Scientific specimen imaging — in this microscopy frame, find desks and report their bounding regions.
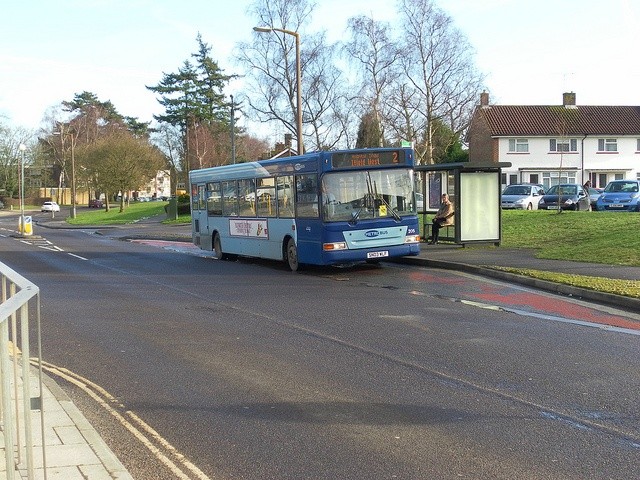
[52,131,77,219]
[251,24,305,157]
[18,143,27,237]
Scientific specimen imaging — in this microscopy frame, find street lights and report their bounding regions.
[432,213,446,224]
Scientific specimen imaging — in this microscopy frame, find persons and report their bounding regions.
[312,184,338,219]
[429,194,454,244]
[582,180,591,195]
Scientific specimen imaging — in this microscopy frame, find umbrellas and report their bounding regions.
[428,241,438,245]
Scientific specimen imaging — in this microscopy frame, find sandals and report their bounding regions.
[188,146,421,273]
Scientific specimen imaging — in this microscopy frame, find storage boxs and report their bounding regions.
[425,222,455,242]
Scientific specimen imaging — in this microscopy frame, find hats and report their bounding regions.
[538,183,592,212]
[40,201,61,213]
[584,187,601,210]
[595,180,640,211]
[90,199,104,209]
[501,184,544,211]
